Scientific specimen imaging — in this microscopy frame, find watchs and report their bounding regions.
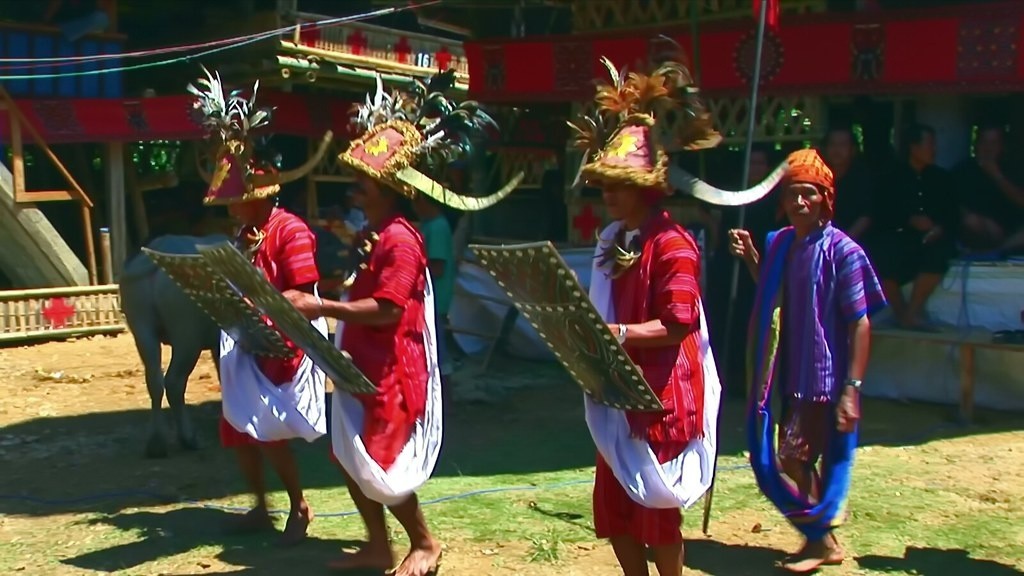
[616,324,626,345]
[845,380,864,392]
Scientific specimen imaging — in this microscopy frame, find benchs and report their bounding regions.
[870,253,1024,333]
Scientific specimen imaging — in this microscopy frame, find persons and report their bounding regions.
[580,113,723,576]
[728,148,887,573]
[204,140,330,547]
[739,118,1024,331]
[282,118,442,576]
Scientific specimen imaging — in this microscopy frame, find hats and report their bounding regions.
[562,55,788,205]
[185,62,333,206]
[781,146,836,225]
[339,70,525,212]
